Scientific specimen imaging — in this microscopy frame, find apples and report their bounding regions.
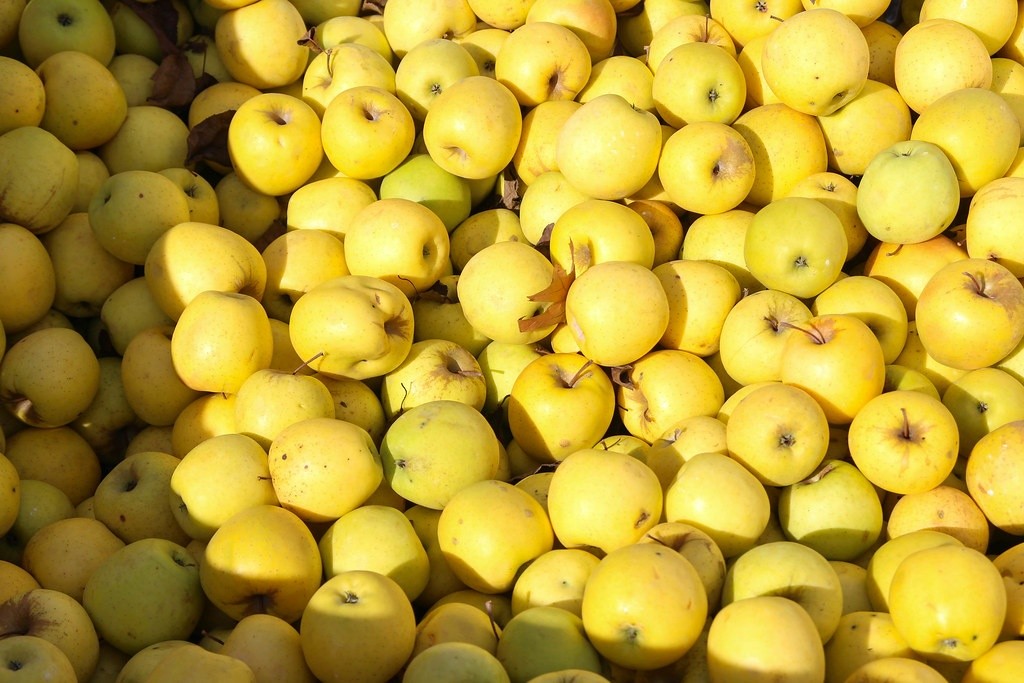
[0,0,1024,683]
